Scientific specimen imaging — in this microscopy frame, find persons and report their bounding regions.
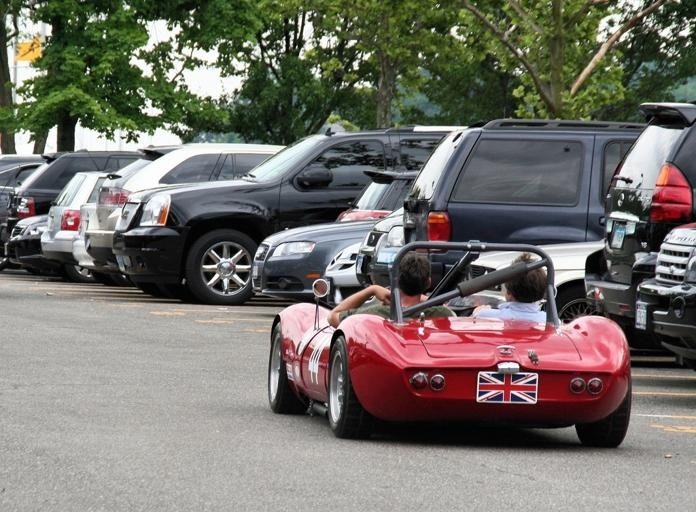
[470,252,562,324]
[326,253,457,327]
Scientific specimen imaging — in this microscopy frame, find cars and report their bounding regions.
[266,244,631,448]
[78,140,289,299]
[0,146,141,284]
[248,167,427,305]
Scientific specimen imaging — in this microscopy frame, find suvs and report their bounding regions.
[404,118,677,359]
[585,101,696,373]
[357,118,677,359]
[110,124,472,307]
